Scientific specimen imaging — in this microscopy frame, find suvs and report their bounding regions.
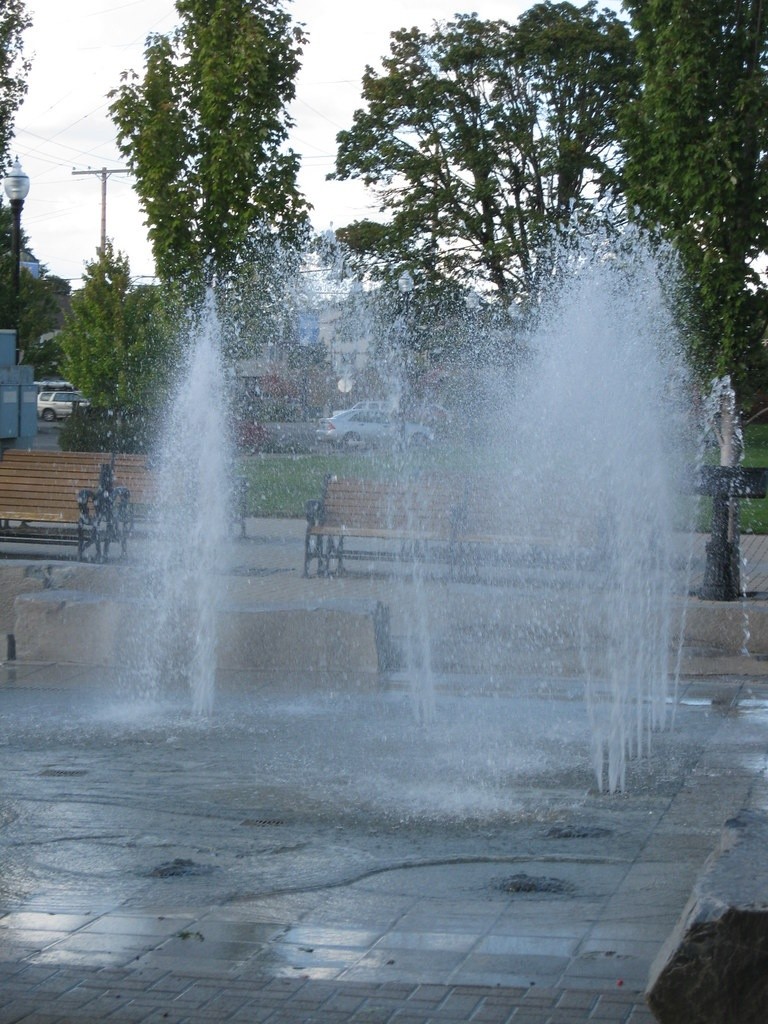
[37,391,115,423]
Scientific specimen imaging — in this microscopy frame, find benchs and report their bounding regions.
[456,477,614,590]
[0,448,116,562]
[303,474,459,577]
[95,453,225,569]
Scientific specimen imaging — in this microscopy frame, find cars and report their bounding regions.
[315,408,434,449]
[333,401,398,419]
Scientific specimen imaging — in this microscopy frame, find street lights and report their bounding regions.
[3,154,31,364]
[396,270,412,451]
